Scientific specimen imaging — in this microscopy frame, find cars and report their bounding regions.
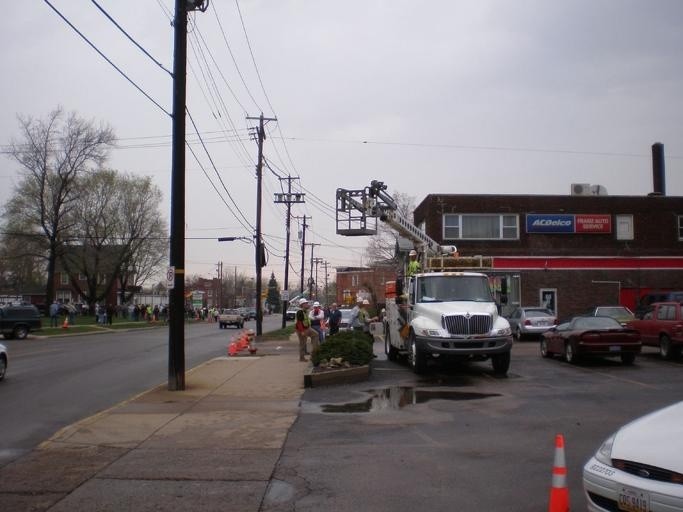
[326,309,355,336]
[624,299,683,361]
[236,308,251,321]
[248,307,256,320]
[0,342,10,384]
[503,305,561,341]
[578,397,683,511]
[589,304,636,327]
[634,291,683,308]
[35,300,77,316]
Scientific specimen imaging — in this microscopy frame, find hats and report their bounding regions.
[313,302,321,307]
[357,297,363,302]
[363,300,370,305]
[299,298,309,305]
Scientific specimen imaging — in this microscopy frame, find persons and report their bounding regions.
[407,249,422,276]
[49,300,220,328]
[295,297,379,362]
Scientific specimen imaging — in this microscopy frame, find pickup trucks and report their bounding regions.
[216,308,245,329]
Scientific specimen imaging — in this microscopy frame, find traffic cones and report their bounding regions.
[225,327,249,357]
[61,316,69,329]
[548,432,571,512]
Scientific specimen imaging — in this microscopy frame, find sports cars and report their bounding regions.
[540,315,642,364]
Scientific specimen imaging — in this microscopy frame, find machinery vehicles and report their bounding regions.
[335,179,513,376]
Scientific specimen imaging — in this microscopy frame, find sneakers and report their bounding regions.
[300,358,308,362]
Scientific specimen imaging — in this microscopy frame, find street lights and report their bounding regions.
[214,235,263,337]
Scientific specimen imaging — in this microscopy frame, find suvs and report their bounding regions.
[286,305,299,320]
[0,300,44,343]
